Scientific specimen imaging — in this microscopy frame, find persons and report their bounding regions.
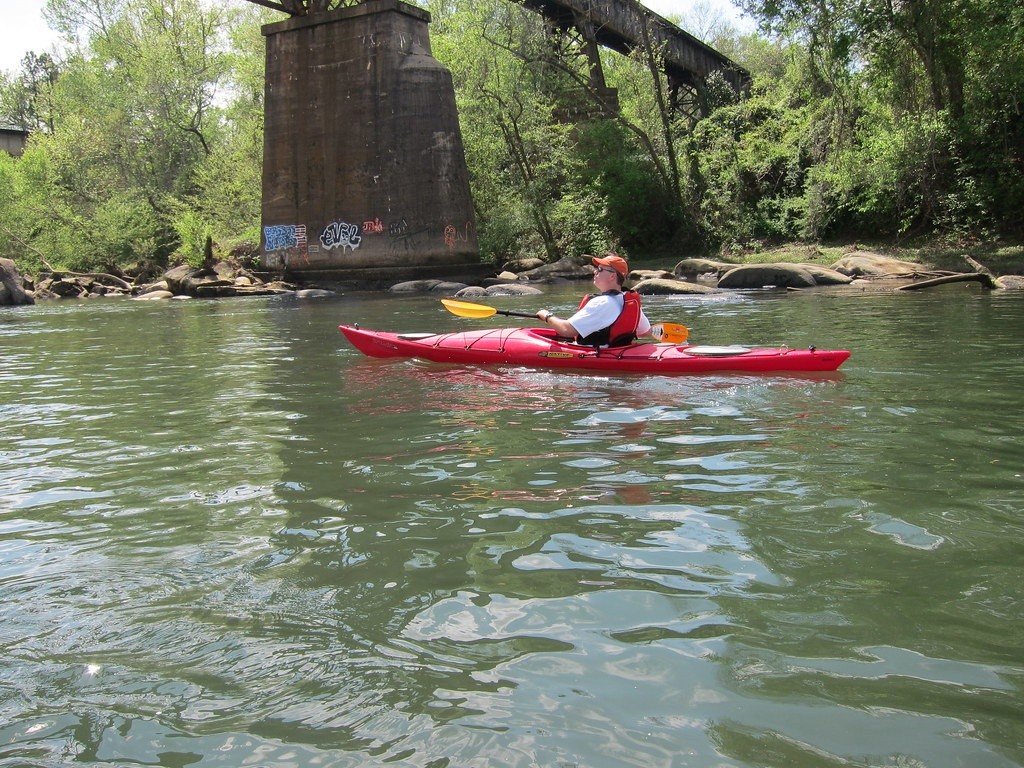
[536,256,654,350]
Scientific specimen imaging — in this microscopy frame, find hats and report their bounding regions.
[592,255,628,278]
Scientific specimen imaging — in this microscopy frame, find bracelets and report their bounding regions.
[546,312,554,324]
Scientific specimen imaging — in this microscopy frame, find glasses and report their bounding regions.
[596,267,616,273]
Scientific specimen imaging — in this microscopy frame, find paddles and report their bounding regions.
[441,298,689,345]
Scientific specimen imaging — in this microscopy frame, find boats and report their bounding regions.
[335,323,851,374]
